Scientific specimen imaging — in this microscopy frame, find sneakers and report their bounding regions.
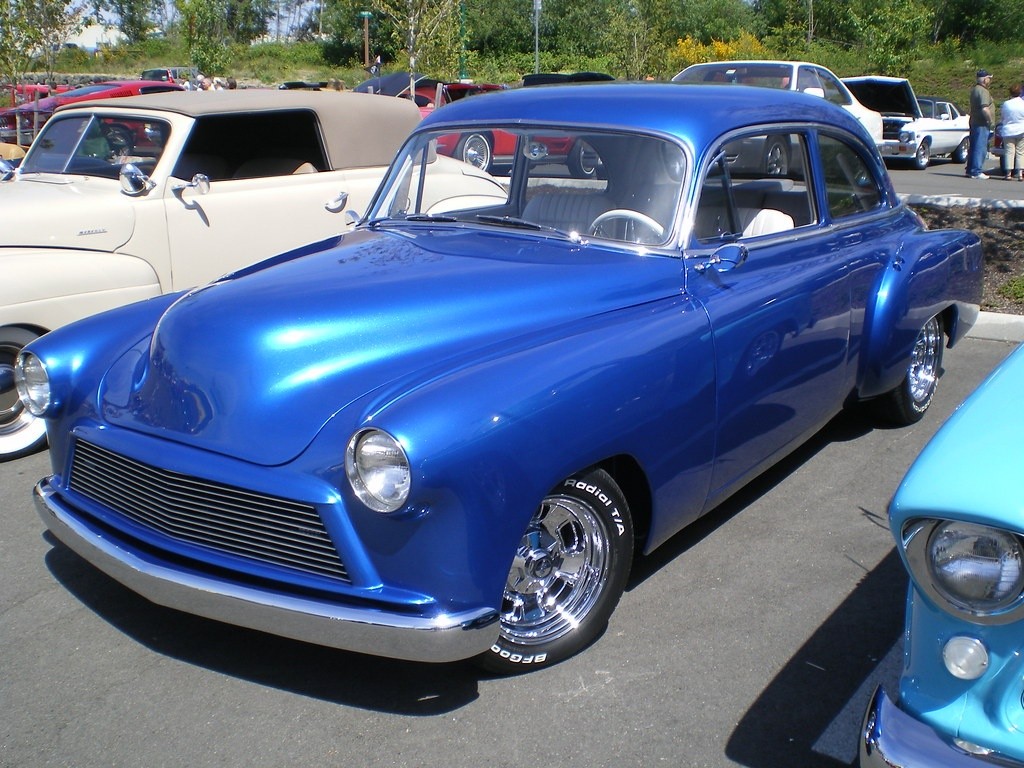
[966,173,990,179]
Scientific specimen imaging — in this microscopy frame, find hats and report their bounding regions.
[977,69,993,78]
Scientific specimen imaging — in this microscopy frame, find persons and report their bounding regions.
[193,75,223,91]
[966,70,995,180]
[225,76,238,89]
[1000,84,1024,181]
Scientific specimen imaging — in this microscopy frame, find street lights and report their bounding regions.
[359,11,374,80]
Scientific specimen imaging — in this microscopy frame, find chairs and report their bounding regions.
[520,193,616,237]
[694,206,795,240]
[187,153,231,177]
[231,157,301,179]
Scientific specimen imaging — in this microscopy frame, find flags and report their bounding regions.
[361,56,381,75]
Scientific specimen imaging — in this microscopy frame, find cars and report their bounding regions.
[991,95,1024,175]
[0,84,509,460]
[0,81,188,156]
[838,76,995,171]
[13,80,983,673]
[856,343,1024,768]
[596,61,883,187]
[139,67,208,90]
[352,72,597,179]
[47,44,79,66]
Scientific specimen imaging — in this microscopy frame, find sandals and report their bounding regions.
[1005,175,1023,182]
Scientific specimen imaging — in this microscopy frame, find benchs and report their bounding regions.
[699,178,873,227]
